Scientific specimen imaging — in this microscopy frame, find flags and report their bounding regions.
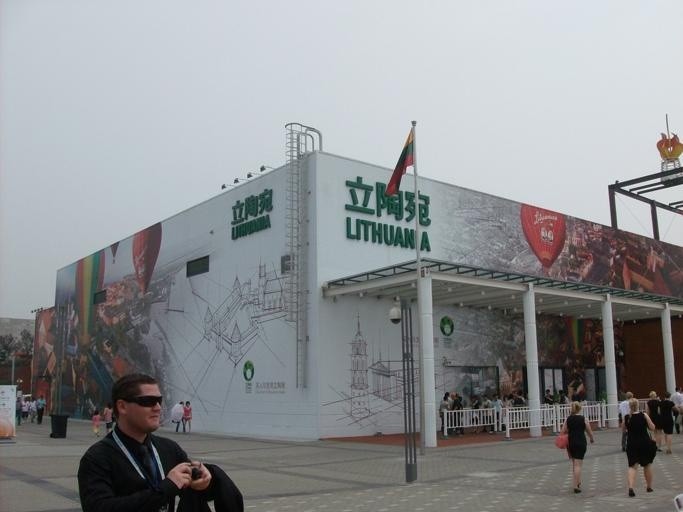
[384,128,413,195]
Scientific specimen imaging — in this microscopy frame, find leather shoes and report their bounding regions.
[657,448,663,452]
[577,484,579,488]
[666,449,672,454]
[574,488,581,493]
[647,487,653,492]
[629,487,635,496]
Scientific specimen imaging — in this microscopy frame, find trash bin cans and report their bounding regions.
[49,414,69,438]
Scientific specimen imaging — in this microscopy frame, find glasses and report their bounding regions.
[123,395,163,408]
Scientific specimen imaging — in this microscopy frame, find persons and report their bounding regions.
[76,373,244,512]
[29,398,38,424]
[37,395,46,424]
[658,392,680,454]
[622,398,656,498]
[170,401,184,432]
[646,391,663,452]
[440,389,578,436]
[671,403,683,427]
[21,397,30,424]
[670,386,683,434]
[619,391,633,451]
[16,395,22,425]
[561,400,594,494]
[102,401,114,433]
[91,409,101,436]
[182,401,193,433]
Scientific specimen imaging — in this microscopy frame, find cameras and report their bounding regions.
[188,465,202,480]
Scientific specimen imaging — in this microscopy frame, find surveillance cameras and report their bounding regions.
[388,306,402,325]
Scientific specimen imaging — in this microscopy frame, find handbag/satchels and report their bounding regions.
[648,438,658,459]
[555,433,569,450]
